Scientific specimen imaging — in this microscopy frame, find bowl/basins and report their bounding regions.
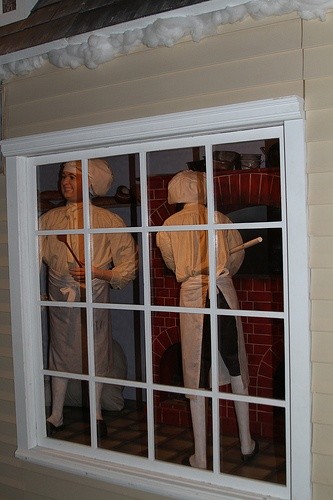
[187,150,263,174]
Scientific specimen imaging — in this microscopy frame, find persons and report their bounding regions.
[37,158,136,440]
[153,170,258,472]
